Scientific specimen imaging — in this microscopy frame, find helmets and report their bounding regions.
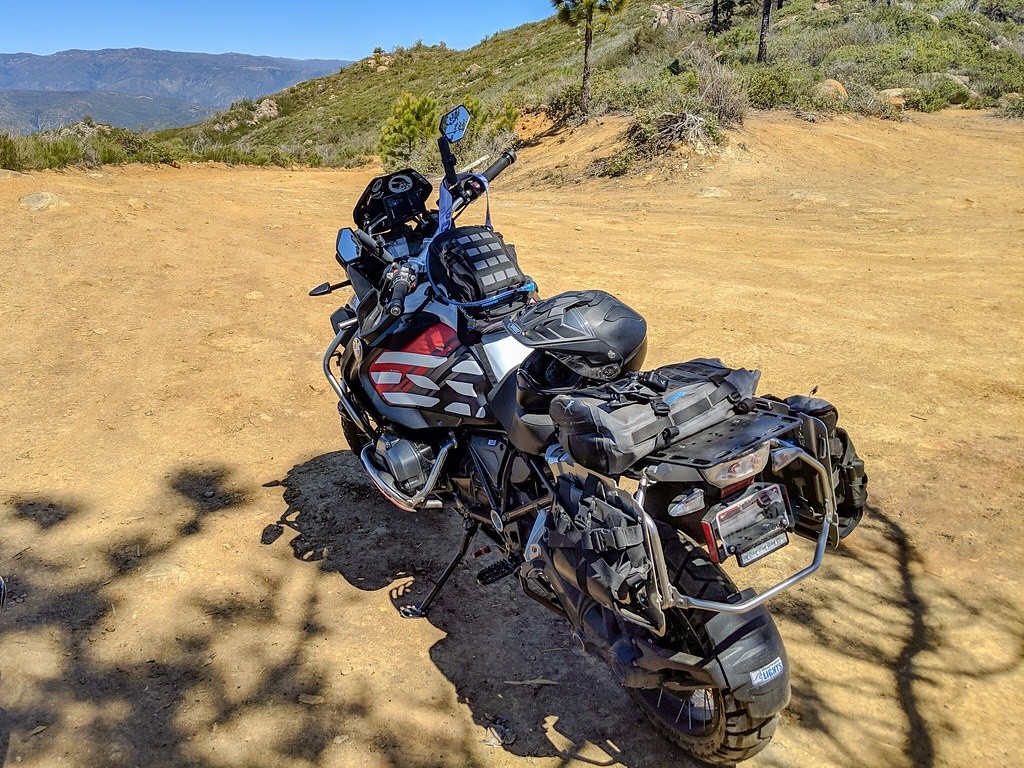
[503,290,648,415]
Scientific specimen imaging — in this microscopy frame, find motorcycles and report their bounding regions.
[310,104,840,766]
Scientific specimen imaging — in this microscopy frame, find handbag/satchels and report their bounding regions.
[549,358,761,475]
[762,394,869,540]
[541,478,653,631]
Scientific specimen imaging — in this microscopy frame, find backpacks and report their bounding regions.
[426,176,539,338]
